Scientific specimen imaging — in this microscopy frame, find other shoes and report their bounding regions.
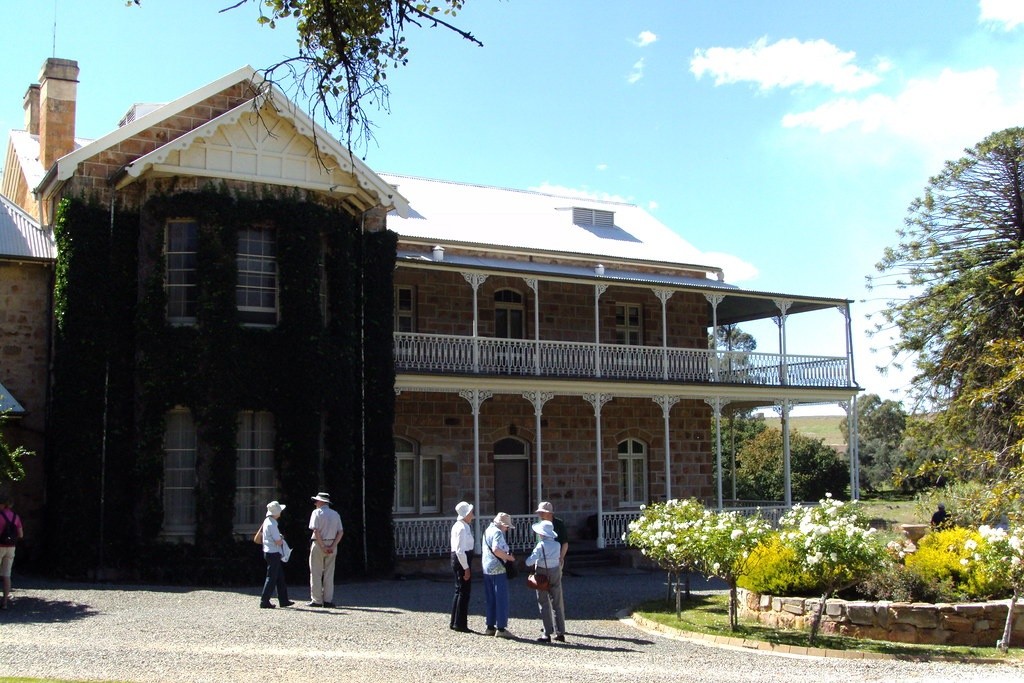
[555,635,564,641]
[306,602,322,607]
[280,601,294,607]
[323,602,336,608]
[495,629,516,638]
[537,636,551,643]
[449,625,474,633]
[0,599,7,609]
[486,627,496,635]
[260,601,276,608]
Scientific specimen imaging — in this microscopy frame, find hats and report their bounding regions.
[535,502,554,514]
[532,520,558,538]
[454,502,473,521]
[310,492,332,504]
[494,512,515,529]
[266,501,286,516]
[938,502,945,509]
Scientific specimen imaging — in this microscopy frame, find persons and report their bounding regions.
[0,497,24,610]
[261,501,294,608]
[481,513,516,639]
[525,502,568,643]
[309,492,344,608]
[930,502,955,532]
[450,501,475,633]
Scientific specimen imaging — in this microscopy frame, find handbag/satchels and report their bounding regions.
[528,574,550,592]
[254,524,263,544]
[505,560,519,579]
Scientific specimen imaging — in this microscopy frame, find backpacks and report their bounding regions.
[0,511,18,546]
